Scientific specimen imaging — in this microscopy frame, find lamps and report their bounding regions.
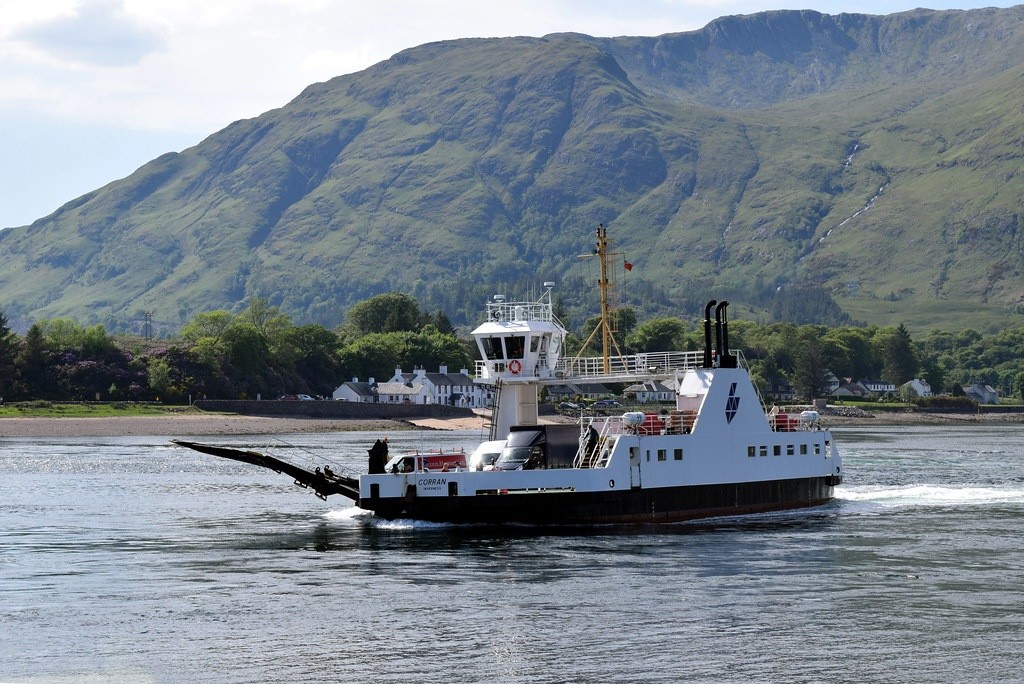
[555,371,564,380]
[649,367,658,374]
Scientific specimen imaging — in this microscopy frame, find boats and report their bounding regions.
[168,220,844,532]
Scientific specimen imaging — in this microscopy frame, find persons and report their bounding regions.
[583,425,599,456]
[391,460,462,473]
[768,402,779,428]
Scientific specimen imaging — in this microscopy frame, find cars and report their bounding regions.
[277,394,349,403]
[559,401,623,410]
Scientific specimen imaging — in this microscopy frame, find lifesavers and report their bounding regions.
[509,360,521,374]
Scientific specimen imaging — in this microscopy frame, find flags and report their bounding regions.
[625,259,634,270]
